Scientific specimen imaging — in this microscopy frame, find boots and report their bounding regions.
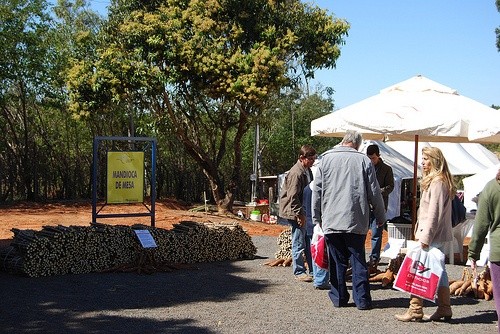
[395,294,424,321]
[423,285,452,321]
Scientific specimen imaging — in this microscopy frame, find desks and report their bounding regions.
[245,204,269,219]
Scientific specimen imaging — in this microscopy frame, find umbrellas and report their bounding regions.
[311,74,500,241]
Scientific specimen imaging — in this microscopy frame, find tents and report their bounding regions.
[278,139,500,221]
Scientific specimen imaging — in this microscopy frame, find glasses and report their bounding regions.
[305,156,314,160]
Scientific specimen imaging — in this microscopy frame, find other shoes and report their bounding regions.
[368,256,381,273]
[296,274,312,282]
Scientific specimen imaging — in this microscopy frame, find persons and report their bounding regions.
[278,144,318,282]
[312,130,387,310]
[312,260,330,290]
[394,147,457,322]
[461,152,500,334]
[345,144,395,276]
[303,180,313,274]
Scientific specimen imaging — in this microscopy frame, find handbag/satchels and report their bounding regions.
[310,223,328,270]
[452,195,466,228]
[392,240,445,303]
